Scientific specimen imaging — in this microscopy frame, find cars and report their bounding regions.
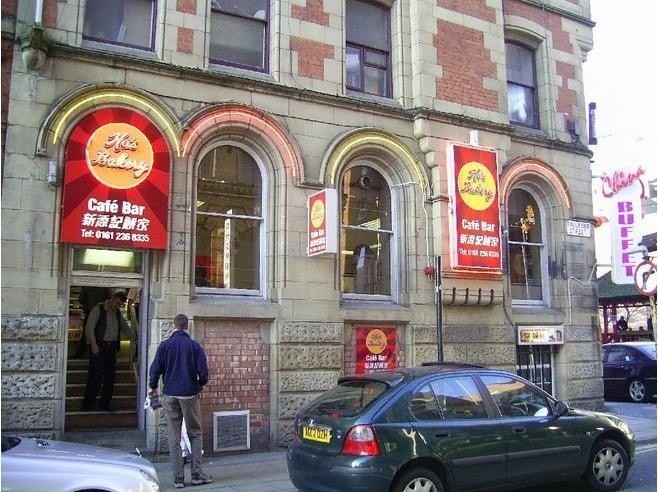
[1,431,161,492]
[602,341,657,401]
[287,361,635,489]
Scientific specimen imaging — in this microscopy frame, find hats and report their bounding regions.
[174,314,188,330]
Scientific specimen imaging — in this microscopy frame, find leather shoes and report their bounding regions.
[101,407,117,411]
[81,398,91,411]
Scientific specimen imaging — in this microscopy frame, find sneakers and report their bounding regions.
[191,472,214,485]
[172,476,185,488]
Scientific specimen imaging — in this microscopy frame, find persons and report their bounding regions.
[148,314,214,488]
[607,315,628,343]
[69,286,138,411]
